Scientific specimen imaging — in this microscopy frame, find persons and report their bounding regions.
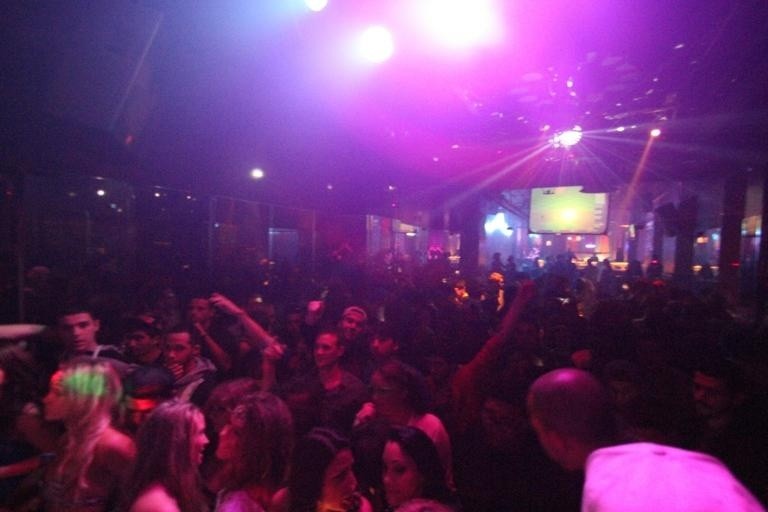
[0,247,768,512]
